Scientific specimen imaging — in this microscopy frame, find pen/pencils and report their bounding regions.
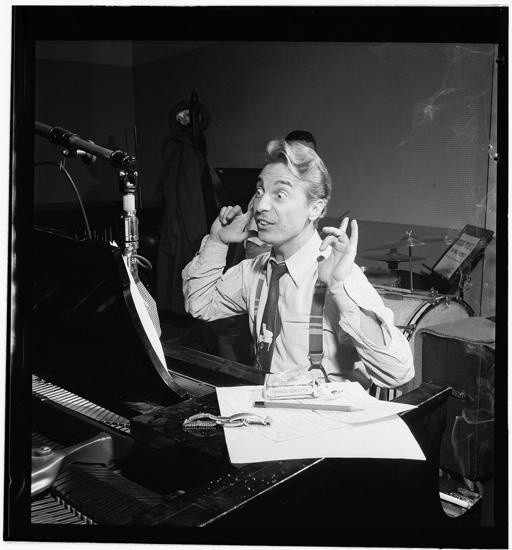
[254,400,363,412]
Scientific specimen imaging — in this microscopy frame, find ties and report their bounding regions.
[254,259,288,374]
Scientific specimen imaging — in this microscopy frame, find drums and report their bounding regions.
[361,271,406,291]
[369,283,477,403]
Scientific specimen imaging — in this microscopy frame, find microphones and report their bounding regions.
[49,125,138,173]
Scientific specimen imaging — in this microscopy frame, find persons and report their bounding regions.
[244,130,318,266]
[182,138,415,388]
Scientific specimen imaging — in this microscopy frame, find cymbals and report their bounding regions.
[360,252,429,267]
[357,236,457,252]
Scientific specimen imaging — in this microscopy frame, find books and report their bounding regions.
[214,382,427,461]
